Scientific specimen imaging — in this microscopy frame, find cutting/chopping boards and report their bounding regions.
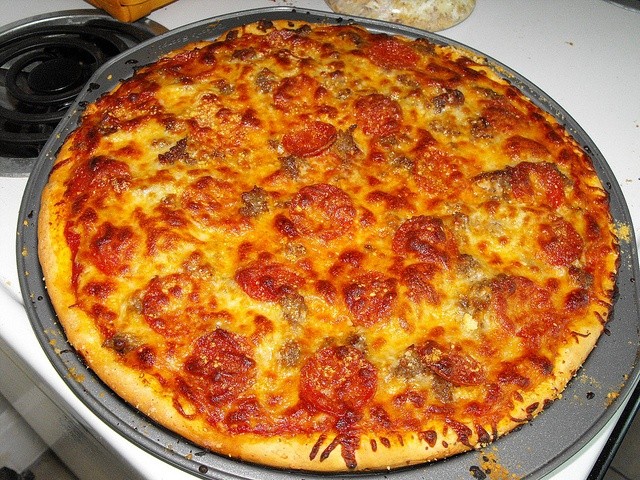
[82,0,173,24]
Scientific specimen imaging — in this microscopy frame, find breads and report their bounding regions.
[323,0,478,33]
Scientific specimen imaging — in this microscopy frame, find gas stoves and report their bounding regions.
[0,8,169,176]
